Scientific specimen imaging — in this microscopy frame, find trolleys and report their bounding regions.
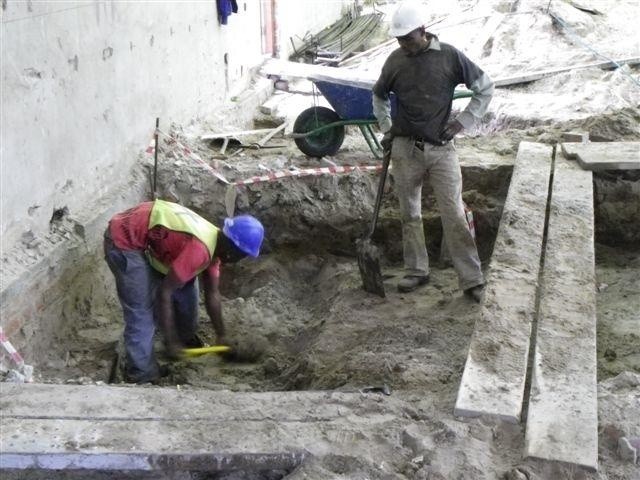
[289,76,473,160]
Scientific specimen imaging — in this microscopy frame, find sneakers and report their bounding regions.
[465,285,484,301]
[179,333,205,350]
[397,273,430,293]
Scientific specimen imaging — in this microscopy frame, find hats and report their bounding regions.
[389,2,433,38]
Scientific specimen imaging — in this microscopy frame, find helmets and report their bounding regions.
[223,213,265,258]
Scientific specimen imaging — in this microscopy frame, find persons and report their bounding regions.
[102,198,267,386]
[371,3,494,302]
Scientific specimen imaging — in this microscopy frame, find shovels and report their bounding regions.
[356,142,391,297]
[213,136,242,154]
